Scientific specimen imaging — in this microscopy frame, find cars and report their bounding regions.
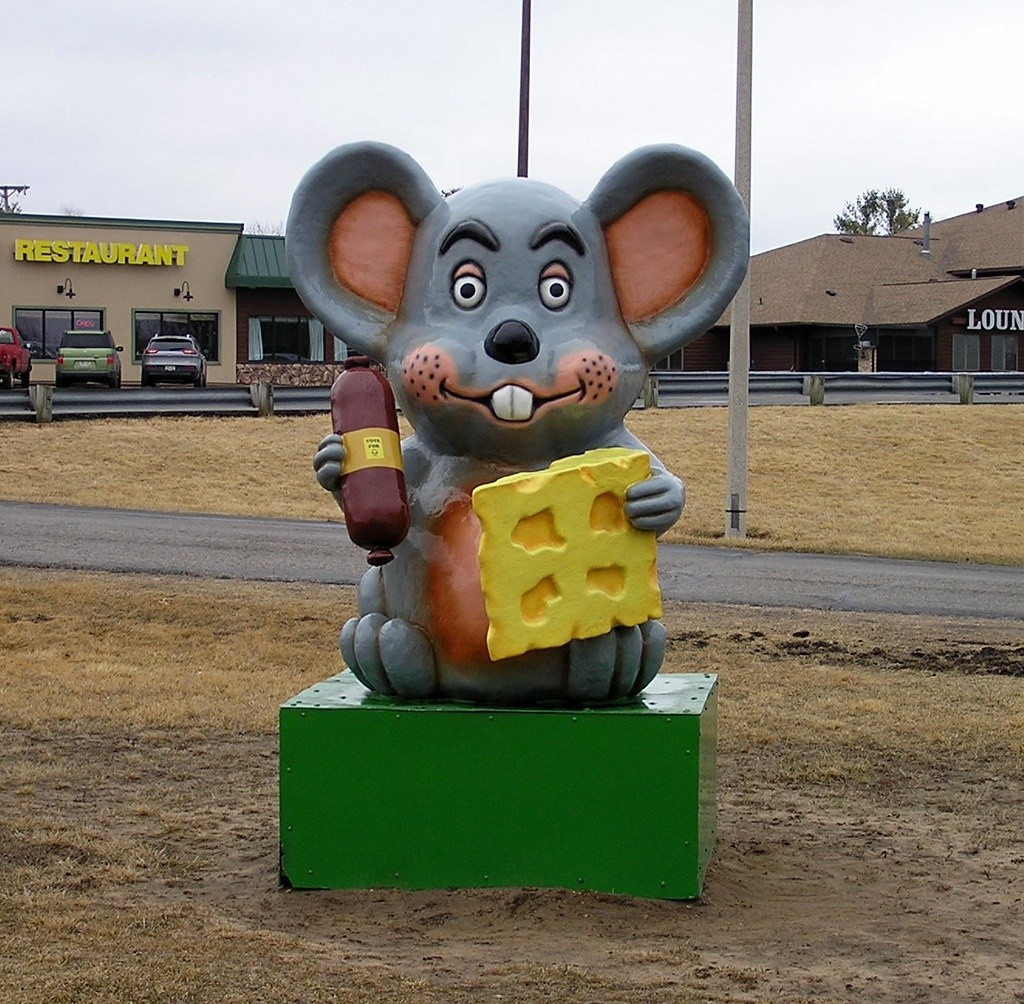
[24,343,57,359]
[262,354,309,363]
[335,350,368,362]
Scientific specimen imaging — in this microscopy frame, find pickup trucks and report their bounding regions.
[0,328,32,390]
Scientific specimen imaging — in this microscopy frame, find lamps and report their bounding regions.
[174,281,194,302]
[57,277,77,300]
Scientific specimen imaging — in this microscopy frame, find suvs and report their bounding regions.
[54,330,124,389]
[139,333,210,387]
